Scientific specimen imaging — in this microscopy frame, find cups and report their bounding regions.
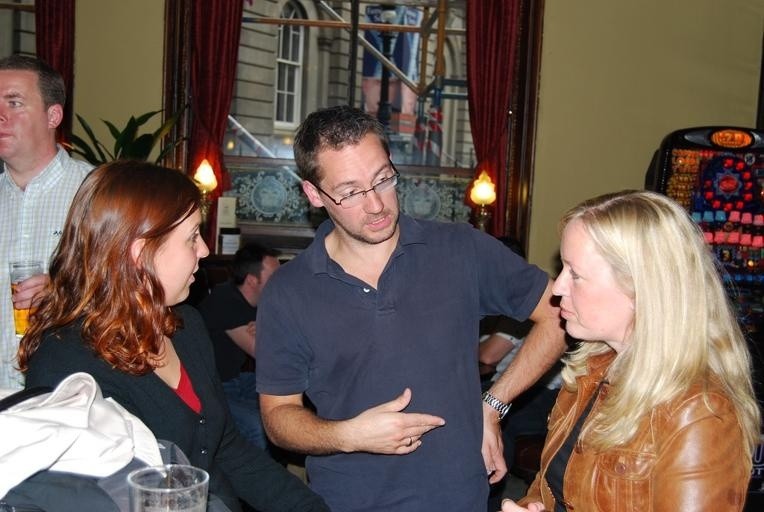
[10,262,46,336]
[127,464,211,512]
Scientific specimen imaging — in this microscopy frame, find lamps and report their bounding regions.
[470,169,496,232]
[192,159,219,236]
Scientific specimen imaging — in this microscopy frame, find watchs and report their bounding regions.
[483,391,512,419]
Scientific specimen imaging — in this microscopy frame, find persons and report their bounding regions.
[0,51,97,399]
[15,158,332,512]
[502,190,763,512]
[256,105,576,511]
[209,245,279,451]
[479,312,528,387]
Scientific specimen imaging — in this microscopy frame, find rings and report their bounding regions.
[406,437,413,446]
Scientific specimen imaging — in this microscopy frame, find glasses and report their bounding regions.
[305,158,400,209]
